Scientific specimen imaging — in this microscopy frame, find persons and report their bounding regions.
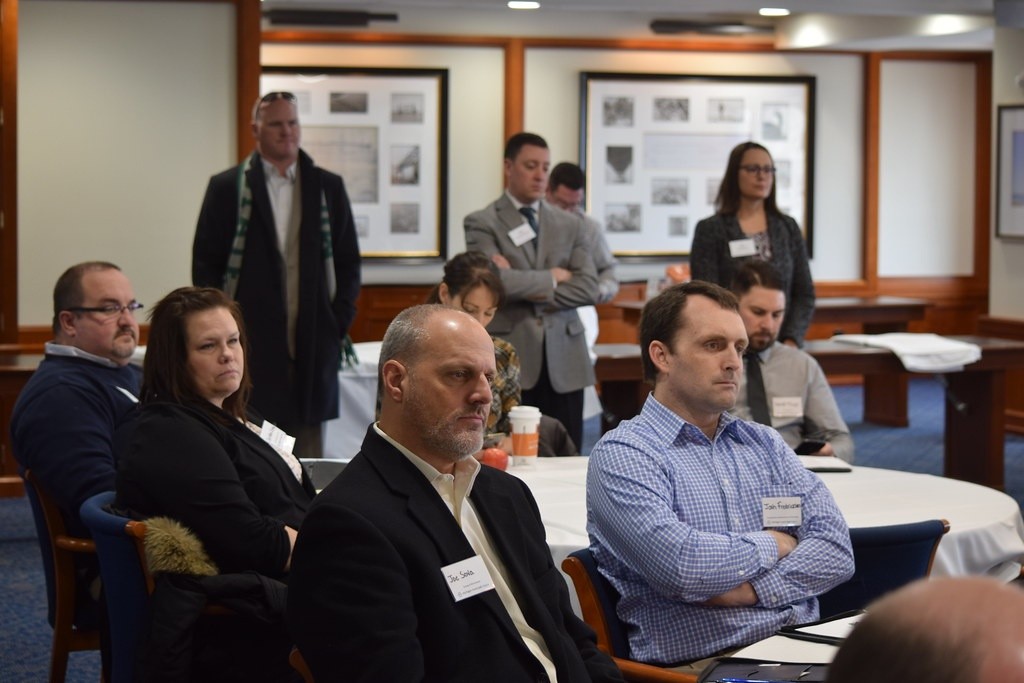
[463,132,600,458]
[10,262,145,632]
[586,281,855,676]
[111,286,316,683]
[426,251,521,457]
[731,263,854,465]
[192,92,360,458]
[288,304,624,683]
[546,161,620,458]
[825,574,1024,683]
[689,141,815,348]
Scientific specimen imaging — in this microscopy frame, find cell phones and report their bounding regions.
[793,439,826,455]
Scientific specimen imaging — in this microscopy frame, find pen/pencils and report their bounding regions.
[717,677,793,683]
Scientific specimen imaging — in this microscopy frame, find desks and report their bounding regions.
[321,297,1023,494]
[501,452,1024,622]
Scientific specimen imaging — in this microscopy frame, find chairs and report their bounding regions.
[19,458,951,683]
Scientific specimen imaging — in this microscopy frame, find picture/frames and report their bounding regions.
[994,105,1024,240]
[578,70,818,266]
[257,65,448,266]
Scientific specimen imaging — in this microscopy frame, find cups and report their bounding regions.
[508,406,542,466]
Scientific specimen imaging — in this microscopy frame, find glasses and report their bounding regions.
[64,302,144,318]
[255,92,296,122]
[739,165,776,175]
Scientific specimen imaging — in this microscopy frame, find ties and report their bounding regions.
[519,208,540,250]
[745,351,771,426]
